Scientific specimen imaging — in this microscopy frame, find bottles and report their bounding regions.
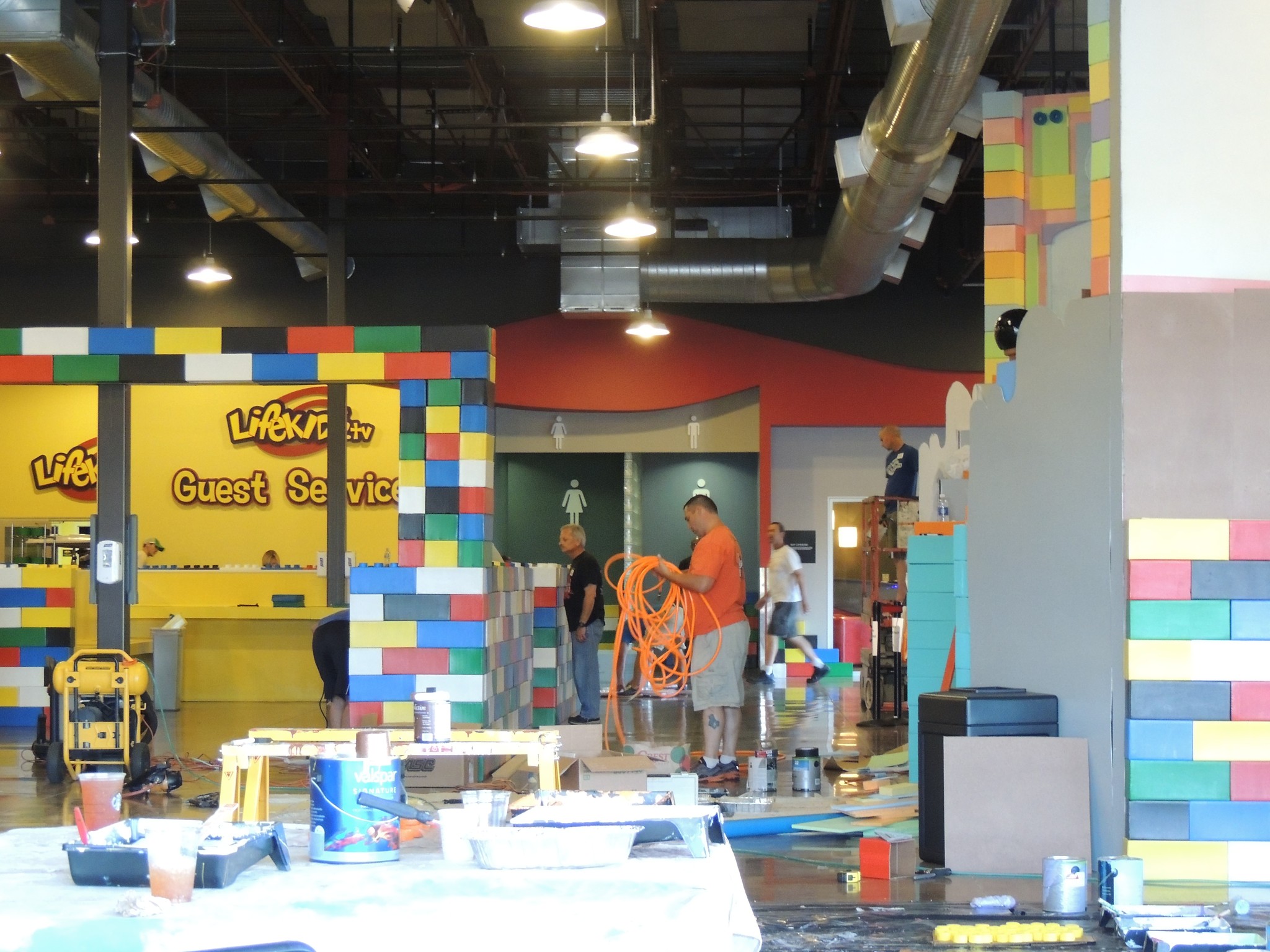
[938,494,948,522]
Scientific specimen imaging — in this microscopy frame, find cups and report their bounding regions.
[460,788,512,827]
[438,807,481,860]
[77,773,127,831]
[146,830,200,901]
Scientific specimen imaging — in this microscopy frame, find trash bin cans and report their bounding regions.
[147,628,181,710]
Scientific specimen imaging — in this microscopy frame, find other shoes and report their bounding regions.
[600,684,624,698]
[617,683,644,698]
[568,715,600,724]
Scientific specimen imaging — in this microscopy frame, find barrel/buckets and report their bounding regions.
[308,755,409,864]
[748,756,778,791]
[1096,856,1144,909]
[1042,855,1087,914]
[791,756,821,791]
[411,701,451,743]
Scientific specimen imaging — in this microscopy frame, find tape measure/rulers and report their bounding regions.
[836,868,861,882]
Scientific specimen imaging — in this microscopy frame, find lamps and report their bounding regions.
[604,48,657,239]
[81,225,141,249]
[574,0,640,160]
[624,301,671,340]
[186,214,234,286]
[837,502,859,550]
[522,0,607,33]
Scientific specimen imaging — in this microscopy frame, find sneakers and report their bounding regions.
[690,756,725,783]
[718,755,740,780]
[807,664,829,683]
[746,670,776,685]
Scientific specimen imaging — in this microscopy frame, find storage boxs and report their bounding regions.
[862,778,891,791]
[917,684,1061,864]
[857,836,916,881]
[878,783,918,797]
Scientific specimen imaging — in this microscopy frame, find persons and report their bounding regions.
[137,537,164,568]
[311,609,350,729]
[673,536,701,690]
[746,522,830,685]
[262,550,280,566]
[648,494,750,782]
[879,424,919,604]
[994,309,1027,361]
[559,523,606,725]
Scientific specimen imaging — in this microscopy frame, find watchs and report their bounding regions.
[579,622,586,628]
